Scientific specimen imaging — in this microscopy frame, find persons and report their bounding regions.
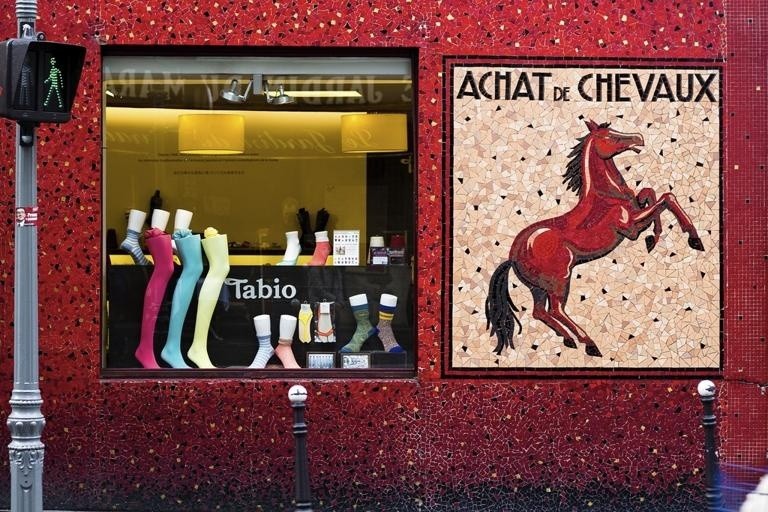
[275,230,303,266]
[151,208,170,232]
[340,293,377,353]
[306,231,332,267]
[374,293,405,352]
[160,229,204,369]
[187,225,230,369]
[171,208,195,231]
[273,314,303,368]
[249,313,275,370]
[134,228,175,368]
[119,209,152,266]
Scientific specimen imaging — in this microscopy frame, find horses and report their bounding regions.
[486,117,706,359]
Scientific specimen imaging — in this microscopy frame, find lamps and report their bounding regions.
[339,111,410,154]
[176,113,246,156]
[217,77,296,107]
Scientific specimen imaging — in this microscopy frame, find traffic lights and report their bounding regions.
[0,38,86,125]
